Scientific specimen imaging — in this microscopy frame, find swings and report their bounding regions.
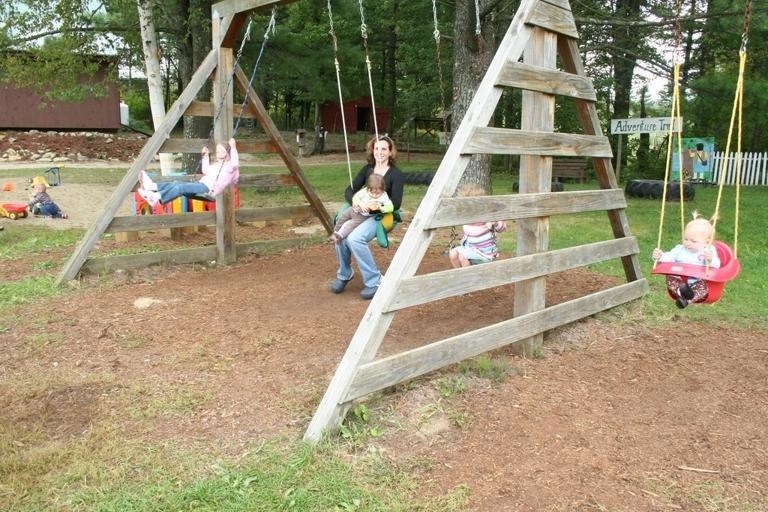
[183,11,276,202]
[432,1,497,265]
[649,21,747,304]
[327,2,402,247]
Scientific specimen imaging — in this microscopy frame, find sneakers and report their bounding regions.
[138,170,153,192]
[676,299,687,309]
[360,286,378,299]
[331,274,354,294]
[137,188,158,207]
[326,232,343,245]
[62,213,69,219]
[679,284,694,299]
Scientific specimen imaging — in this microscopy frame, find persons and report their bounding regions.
[136,137,241,209]
[326,135,406,301]
[327,174,395,243]
[28,175,69,221]
[447,183,508,269]
[651,207,722,310]
